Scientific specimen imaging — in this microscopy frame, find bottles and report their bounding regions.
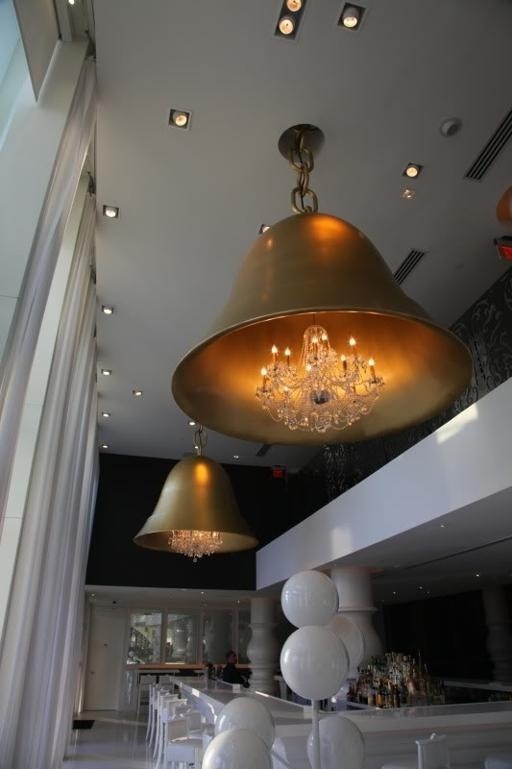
[347,653,446,708]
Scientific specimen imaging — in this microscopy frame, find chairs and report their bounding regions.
[128,671,208,768]
[382,733,449,768]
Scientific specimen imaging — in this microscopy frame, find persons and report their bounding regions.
[221,650,251,688]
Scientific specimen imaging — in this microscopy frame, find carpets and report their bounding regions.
[73,719,95,731]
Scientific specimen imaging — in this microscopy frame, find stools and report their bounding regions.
[483,749,512,769]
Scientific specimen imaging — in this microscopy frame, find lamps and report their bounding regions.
[170,124,475,448]
[134,426,260,557]
[199,569,365,768]
